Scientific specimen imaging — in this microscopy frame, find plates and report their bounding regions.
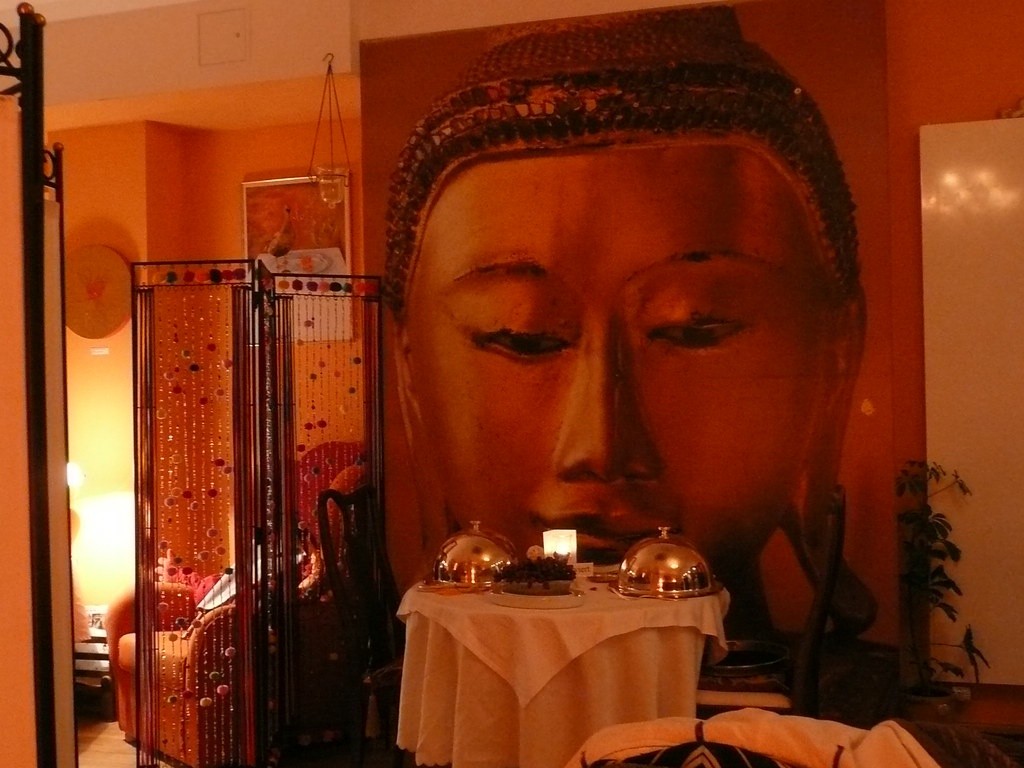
[489,580,573,596]
[699,640,788,679]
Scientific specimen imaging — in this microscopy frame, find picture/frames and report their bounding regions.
[238,177,357,345]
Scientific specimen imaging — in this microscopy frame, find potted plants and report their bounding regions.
[895,460,991,706]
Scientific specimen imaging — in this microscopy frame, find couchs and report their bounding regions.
[105,436,370,768]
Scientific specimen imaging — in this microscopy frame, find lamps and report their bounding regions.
[542,530,577,565]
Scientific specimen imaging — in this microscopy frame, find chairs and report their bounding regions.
[318,485,403,768]
[698,482,850,715]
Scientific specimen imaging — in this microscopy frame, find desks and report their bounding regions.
[396,578,730,768]
